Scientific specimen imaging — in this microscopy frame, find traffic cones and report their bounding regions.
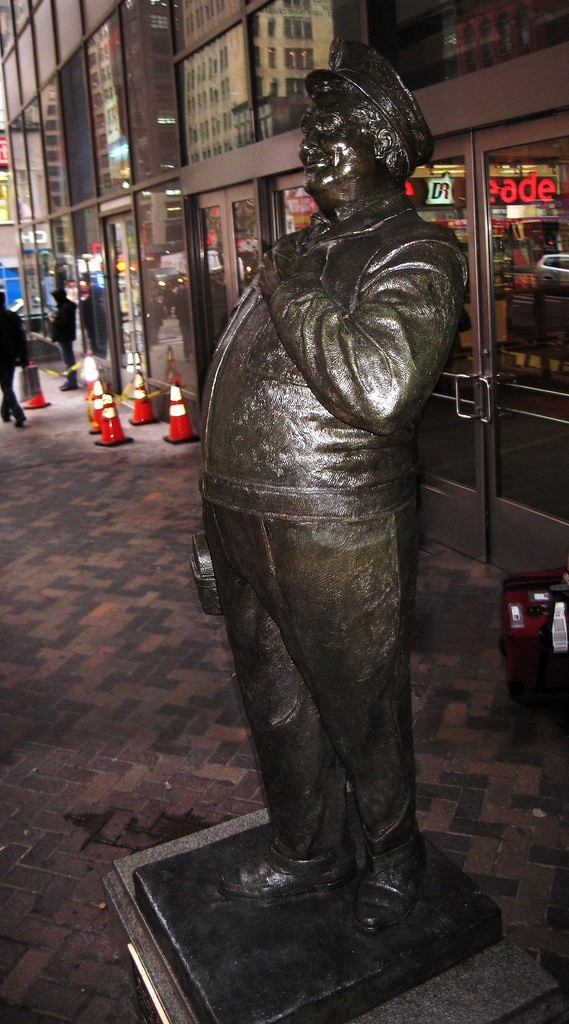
[91,383,134,447]
[88,381,108,433]
[164,378,200,444]
[23,360,51,409]
[130,369,162,425]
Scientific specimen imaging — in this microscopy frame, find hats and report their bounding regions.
[51,290,66,296]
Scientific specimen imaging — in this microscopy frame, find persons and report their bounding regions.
[0,292,27,426]
[198,40,468,933]
[143,269,195,362]
[83,286,109,361]
[49,289,79,391]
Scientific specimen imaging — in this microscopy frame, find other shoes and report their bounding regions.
[15,416,26,427]
[3,416,10,421]
[59,384,78,391]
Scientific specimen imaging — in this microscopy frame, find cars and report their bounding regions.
[144,266,189,304]
[532,253,569,290]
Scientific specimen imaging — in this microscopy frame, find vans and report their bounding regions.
[160,250,261,286]
